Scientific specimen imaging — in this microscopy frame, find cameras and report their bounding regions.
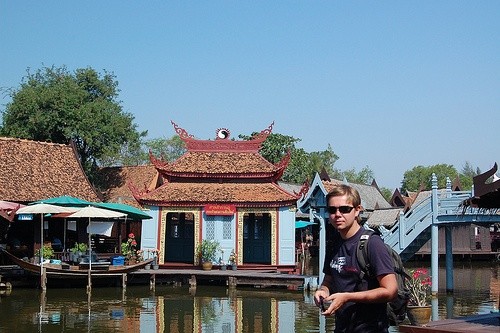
[318,296,329,311]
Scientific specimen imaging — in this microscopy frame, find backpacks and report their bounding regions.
[328,226,412,327]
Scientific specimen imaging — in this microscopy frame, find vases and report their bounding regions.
[407,305,432,326]
[144,263,151,270]
[153,264,160,270]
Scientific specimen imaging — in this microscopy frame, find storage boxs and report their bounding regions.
[111,256,125,266]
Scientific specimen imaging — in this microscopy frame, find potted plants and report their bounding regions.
[36,245,55,264]
[195,239,237,271]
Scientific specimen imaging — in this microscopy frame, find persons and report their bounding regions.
[52,236,61,251]
[314,185,398,333]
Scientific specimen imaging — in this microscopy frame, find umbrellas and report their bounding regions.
[295,220,318,258]
[15,195,153,270]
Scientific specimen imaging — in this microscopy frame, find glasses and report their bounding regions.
[328,206,357,214]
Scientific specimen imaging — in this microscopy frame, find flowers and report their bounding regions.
[401,269,432,308]
[122,233,138,259]
[147,249,160,266]
